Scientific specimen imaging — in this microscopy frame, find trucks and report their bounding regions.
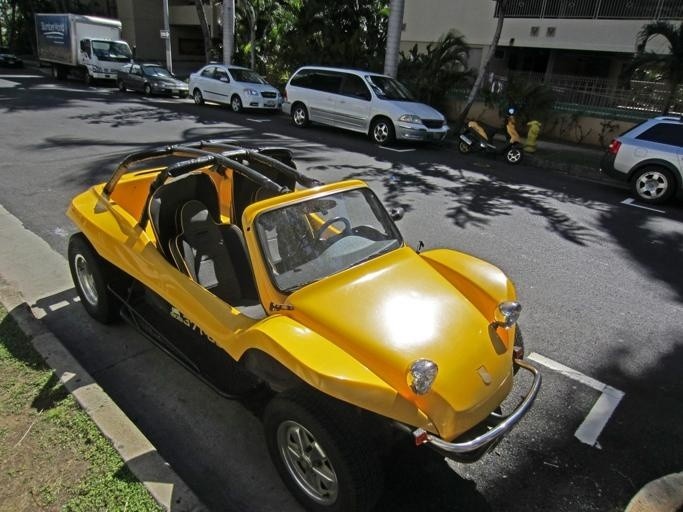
[35,13,132,85]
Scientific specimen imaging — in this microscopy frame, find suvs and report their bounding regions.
[604,117,682,203]
[67,140,544,512]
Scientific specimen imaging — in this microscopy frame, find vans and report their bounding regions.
[284,66,450,147]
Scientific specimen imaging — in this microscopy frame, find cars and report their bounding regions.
[189,63,284,113]
[118,63,190,97]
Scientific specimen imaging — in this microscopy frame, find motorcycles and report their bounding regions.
[459,101,523,164]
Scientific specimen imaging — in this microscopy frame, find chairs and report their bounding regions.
[167,184,320,321]
